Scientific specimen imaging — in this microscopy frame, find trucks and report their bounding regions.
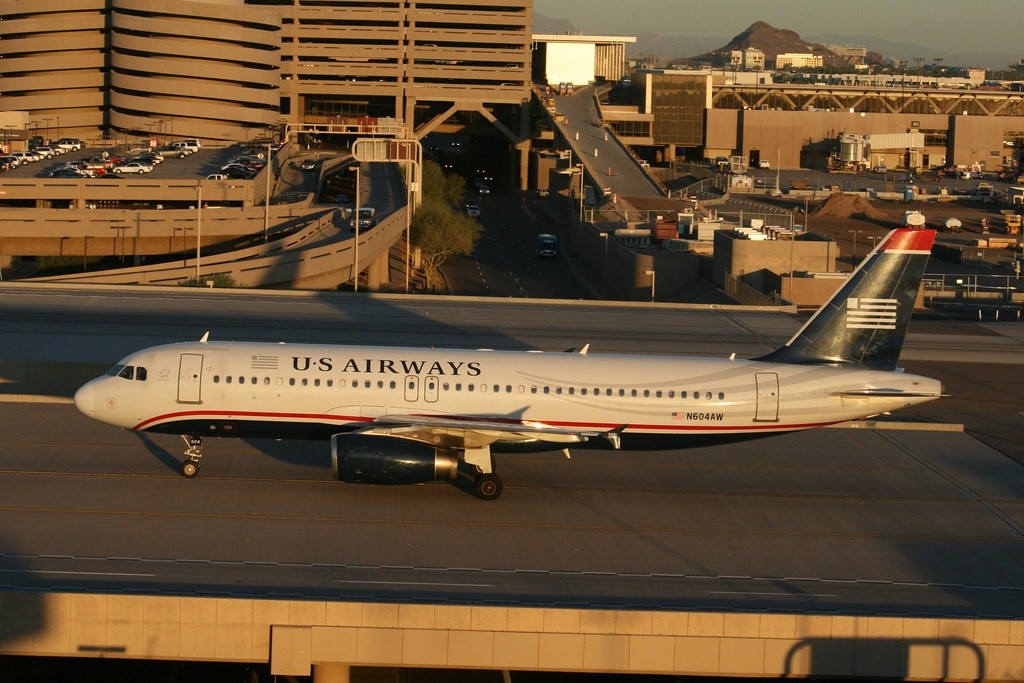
[537,233,559,259]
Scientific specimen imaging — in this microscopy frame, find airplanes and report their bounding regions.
[74,229,954,501]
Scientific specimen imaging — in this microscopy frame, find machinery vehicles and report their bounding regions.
[900,173,914,184]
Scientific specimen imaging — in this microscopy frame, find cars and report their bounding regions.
[206,134,283,183]
[758,160,769,168]
[302,159,314,170]
[51,136,201,183]
[430,140,494,218]
[0,136,80,174]
[716,156,728,165]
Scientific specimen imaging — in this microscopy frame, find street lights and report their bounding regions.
[576,163,584,223]
[79,120,175,175]
[224,123,286,179]
[565,150,571,202]
[2,116,60,157]
[646,270,655,302]
[600,233,608,299]
[350,165,359,290]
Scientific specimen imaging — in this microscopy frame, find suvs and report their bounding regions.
[351,208,375,230]
